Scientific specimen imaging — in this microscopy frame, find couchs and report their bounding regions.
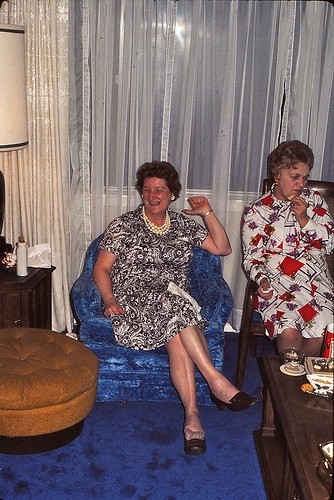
[70,231,234,405]
[0,327,99,455]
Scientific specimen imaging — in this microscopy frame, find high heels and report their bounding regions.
[211,392,258,411]
[183,430,207,454]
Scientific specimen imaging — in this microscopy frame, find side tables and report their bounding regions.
[0,261,57,330]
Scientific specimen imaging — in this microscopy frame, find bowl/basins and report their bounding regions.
[307,374,334,394]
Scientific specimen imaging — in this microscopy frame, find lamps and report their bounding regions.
[0,21,30,261]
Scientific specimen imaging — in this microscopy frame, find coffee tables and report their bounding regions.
[251,350,334,500]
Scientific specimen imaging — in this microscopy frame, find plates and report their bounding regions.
[307,356,334,376]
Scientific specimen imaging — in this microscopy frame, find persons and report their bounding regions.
[242,140,334,357]
[92,161,258,455]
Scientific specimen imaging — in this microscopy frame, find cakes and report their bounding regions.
[304,356,334,380]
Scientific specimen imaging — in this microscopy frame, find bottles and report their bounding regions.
[16,239,28,277]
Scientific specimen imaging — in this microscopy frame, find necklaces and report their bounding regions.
[142,206,171,235]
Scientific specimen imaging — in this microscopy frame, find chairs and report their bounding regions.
[235,178,334,390]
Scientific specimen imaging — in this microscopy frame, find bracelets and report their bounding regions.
[104,298,114,303]
[202,209,213,217]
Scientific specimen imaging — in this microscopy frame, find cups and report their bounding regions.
[321,330,334,358]
[283,348,305,370]
